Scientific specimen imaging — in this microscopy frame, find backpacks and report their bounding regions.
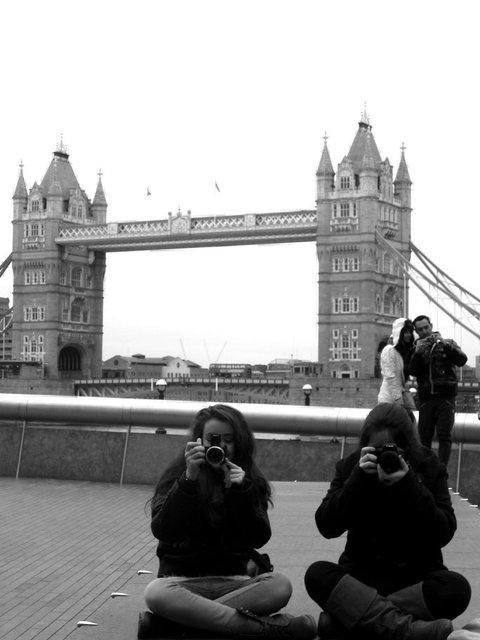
[246,553,270,577]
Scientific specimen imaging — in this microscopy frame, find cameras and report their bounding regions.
[199,434,228,466]
[369,442,408,475]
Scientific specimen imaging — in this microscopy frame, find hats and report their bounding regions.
[392,318,414,345]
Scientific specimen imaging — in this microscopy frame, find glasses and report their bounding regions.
[418,325,429,331]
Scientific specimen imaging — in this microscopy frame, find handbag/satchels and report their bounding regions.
[427,378,458,397]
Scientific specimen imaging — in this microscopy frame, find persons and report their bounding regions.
[405,314,467,477]
[304,402,471,640]
[377,318,416,426]
[136,403,317,640]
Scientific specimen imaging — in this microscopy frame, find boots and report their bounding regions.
[228,607,316,640]
[138,611,161,640]
[318,575,454,639]
